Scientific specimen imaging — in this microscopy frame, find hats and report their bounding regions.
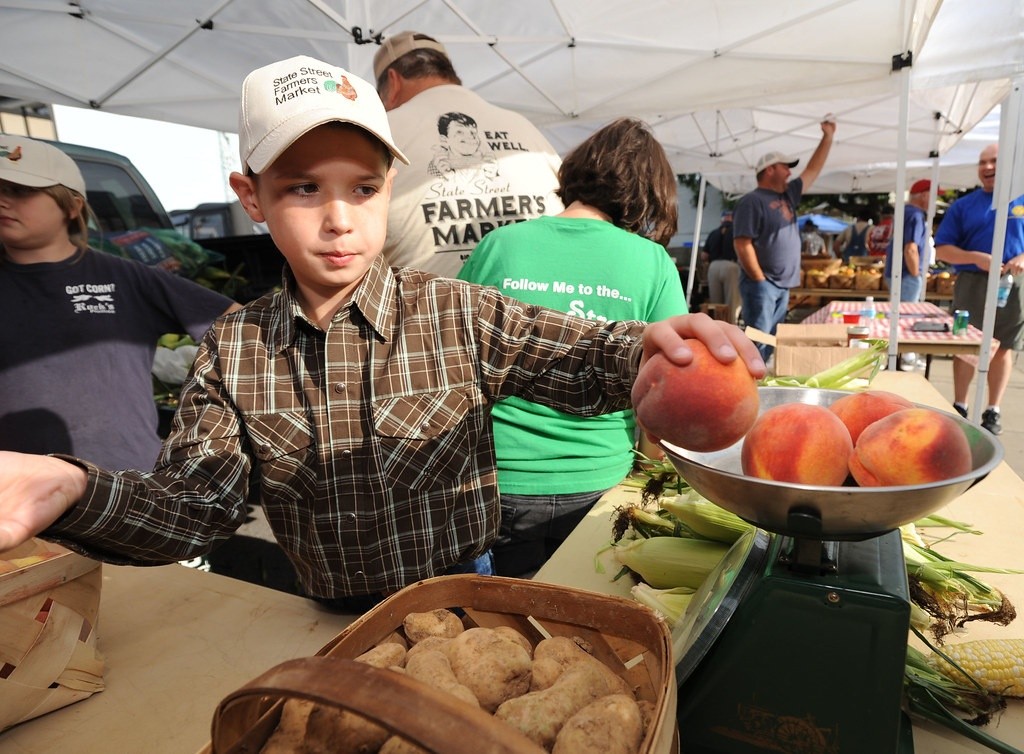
[721,209,732,217]
[239,54,410,176]
[0,134,87,198]
[374,29,451,88]
[755,151,799,175]
[910,179,945,195]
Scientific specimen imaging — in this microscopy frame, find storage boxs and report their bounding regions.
[744,320,875,380]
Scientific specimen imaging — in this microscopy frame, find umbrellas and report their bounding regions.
[797,213,849,233]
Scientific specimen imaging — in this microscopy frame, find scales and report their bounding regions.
[649,385,1004,754]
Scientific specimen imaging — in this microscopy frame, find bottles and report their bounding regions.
[997,270,1013,307]
[859,294,875,335]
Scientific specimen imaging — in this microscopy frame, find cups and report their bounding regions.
[843,313,860,324]
[847,326,870,349]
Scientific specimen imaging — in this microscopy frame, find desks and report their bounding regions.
[1,563,357,754]
[529,367,1024,754]
[791,300,999,381]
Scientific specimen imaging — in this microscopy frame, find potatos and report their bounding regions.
[252,607,644,754]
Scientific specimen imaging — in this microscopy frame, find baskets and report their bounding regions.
[0,537,106,732]
[209,572,677,754]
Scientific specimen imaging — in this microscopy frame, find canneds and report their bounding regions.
[953,310,969,336]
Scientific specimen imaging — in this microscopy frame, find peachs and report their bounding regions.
[629,339,759,453]
[826,390,915,448]
[741,402,853,487]
[849,408,972,488]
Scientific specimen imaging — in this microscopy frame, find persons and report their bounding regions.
[701,210,743,325]
[0,54,767,616]
[373,31,689,578]
[933,143,1024,435]
[0,135,245,572]
[801,219,828,260]
[733,112,836,366]
[679,269,708,315]
[885,180,945,302]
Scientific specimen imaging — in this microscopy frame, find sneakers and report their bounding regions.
[982,408,1003,434]
[953,402,969,419]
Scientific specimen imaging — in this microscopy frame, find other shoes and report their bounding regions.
[900,356,928,371]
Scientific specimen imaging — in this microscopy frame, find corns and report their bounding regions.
[148,229,235,351]
[610,483,1024,698]
